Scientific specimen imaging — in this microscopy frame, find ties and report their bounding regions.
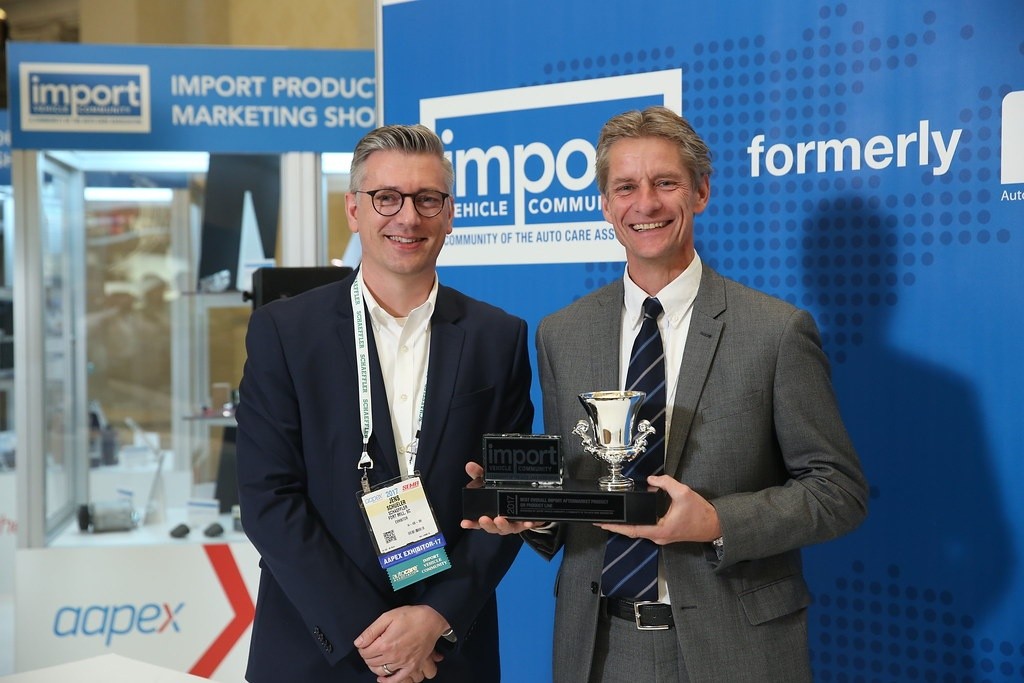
[602,297,664,603]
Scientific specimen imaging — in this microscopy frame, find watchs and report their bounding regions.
[711,536,724,547]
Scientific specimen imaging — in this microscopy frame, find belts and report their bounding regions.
[608,596,674,631]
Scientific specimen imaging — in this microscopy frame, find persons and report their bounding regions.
[457,106,872,683]
[235,122,537,683]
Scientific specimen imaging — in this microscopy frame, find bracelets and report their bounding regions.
[440,628,454,637]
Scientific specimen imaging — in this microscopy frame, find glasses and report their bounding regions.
[355,189,449,218]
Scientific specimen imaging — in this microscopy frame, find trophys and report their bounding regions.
[461,390,668,527]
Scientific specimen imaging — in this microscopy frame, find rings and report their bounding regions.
[383,664,396,676]
